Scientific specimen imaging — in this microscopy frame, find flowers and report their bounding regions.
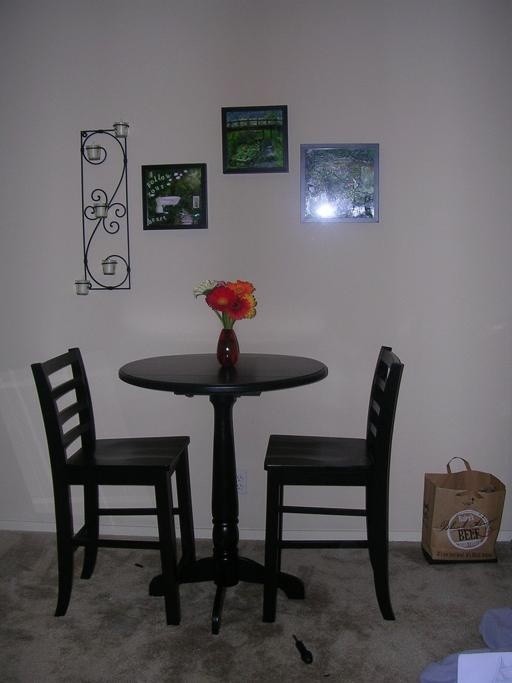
[192,279,257,329]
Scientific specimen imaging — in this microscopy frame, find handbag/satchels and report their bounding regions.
[421,457,507,564]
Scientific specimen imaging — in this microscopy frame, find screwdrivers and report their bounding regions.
[292,634,313,664]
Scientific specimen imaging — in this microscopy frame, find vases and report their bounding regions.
[217,329,239,366]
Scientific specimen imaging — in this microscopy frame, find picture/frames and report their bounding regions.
[221,104,288,174]
[141,162,208,230]
[299,142,380,223]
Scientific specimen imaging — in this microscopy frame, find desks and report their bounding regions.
[117,352,329,633]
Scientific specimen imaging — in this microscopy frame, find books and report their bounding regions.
[457,652,512,683]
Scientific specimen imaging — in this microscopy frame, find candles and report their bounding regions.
[94,200,109,218]
[74,279,90,295]
[102,258,116,274]
[86,141,99,160]
[113,120,128,137]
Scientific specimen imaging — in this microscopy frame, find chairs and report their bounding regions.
[30,343,195,625]
[261,345,404,622]
